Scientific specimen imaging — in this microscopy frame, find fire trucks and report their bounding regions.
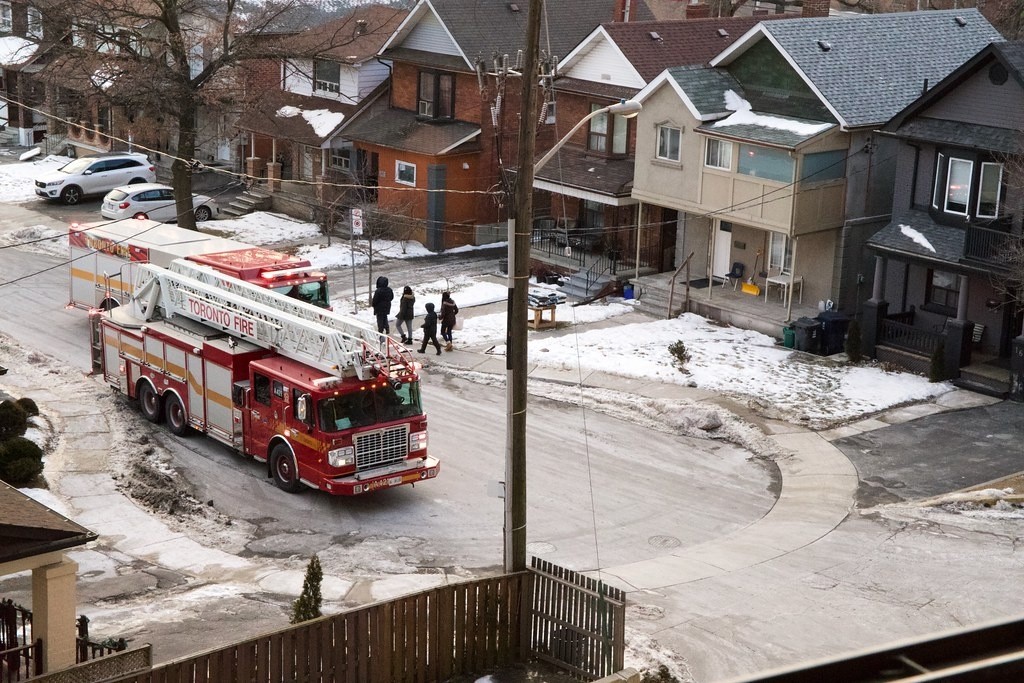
[82,254,442,499]
[64,215,333,316]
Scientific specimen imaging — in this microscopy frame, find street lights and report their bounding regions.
[500,97,644,659]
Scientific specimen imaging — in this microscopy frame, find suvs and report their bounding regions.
[34,150,158,206]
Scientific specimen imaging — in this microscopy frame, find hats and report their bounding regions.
[443,292,451,300]
[404,286,411,294]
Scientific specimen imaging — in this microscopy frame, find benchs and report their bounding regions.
[932,317,985,351]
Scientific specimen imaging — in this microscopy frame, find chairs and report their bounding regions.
[722,262,744,291]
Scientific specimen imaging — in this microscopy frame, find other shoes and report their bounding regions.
[385,326,389,334]
[417,348,425,353]
[444,341,453,351]
[436,350,441,355]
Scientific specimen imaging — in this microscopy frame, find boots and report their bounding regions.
[405,338,413,344]
[401,334,407,343]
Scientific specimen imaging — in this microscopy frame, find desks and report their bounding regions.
[528,305,556,330]
[765,275,803,308]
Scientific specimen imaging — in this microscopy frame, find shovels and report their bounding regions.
[742,251,761,296]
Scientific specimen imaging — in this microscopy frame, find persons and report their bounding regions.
[395,286,416,345]
[417,303,442,356]
[438,292,459,352]
[371,277,393,334]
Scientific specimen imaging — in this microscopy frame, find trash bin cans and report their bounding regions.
[67,143,75,158]
[819,311,849,356]
[623,283,635,299]
[498,258,508,275]
[783,326,795,348]
[789,316,822,354]
[551,629,581,667]
[281,161,292,180]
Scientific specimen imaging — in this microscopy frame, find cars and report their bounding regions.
[100,182,221,223]
